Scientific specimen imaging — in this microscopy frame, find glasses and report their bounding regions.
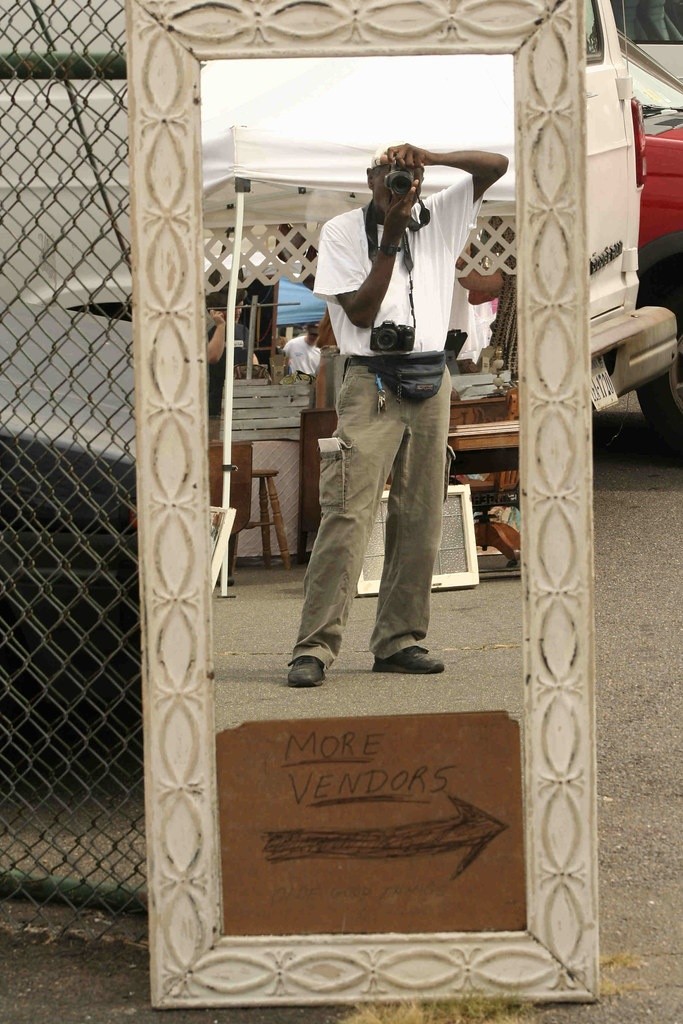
[309,333,319,337]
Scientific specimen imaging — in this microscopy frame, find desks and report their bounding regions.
[448,432,520,580]
[298,397,513,564]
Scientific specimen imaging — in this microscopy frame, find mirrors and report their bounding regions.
[124,0,603,1009]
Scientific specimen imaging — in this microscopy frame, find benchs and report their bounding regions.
[221,379,497,446]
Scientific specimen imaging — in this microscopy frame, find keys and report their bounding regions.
[377,395,387,414]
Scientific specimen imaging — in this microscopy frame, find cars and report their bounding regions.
[1,299,136,789]
[582,0,683,463]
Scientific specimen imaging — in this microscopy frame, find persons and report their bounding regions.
[207,300,259,414]
[456,215,518,380]
[284,322,321,375]
[288,142,511,687]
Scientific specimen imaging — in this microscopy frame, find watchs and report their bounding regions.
[379,244,402,257]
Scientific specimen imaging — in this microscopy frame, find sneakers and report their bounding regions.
[287,656,325,687]
[372,647,445,674]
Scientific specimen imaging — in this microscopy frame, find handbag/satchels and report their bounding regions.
[360,352,446,400]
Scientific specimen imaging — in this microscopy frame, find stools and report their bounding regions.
[232,469,293,576]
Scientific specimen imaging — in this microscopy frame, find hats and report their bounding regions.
[372,143,426,171]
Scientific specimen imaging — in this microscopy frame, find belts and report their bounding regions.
[347,355,367,366]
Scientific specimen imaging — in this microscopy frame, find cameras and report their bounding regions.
[370,322,415,353]
[385,162,414,195]
